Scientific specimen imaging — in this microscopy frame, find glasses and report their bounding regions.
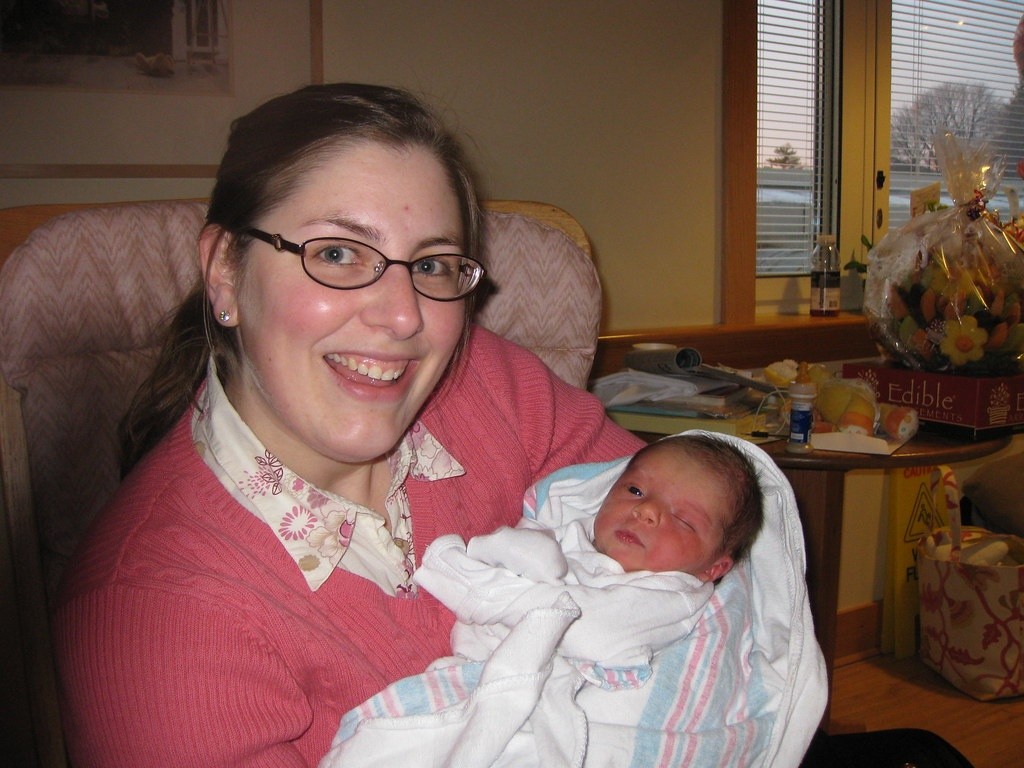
[244,226,488,302]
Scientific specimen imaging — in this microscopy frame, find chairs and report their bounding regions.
[0,197,602,768]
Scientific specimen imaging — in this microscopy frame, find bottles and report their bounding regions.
[809,234,841,316]
[785,362,818,453]
[765,396,779,428]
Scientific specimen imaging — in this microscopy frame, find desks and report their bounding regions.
[629,387,1013,735]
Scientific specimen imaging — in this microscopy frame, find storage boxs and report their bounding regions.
[842,359,1024,445]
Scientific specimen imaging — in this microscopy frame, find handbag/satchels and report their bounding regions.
[916,463,1024,702]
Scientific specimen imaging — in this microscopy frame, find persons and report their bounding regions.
[54,80,985,768]
[326,425,828,768]
